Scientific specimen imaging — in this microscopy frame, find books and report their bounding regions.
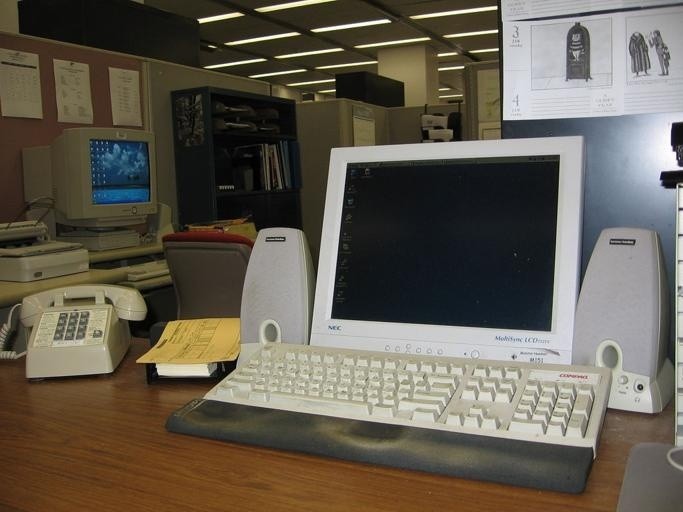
[233,141,292,190]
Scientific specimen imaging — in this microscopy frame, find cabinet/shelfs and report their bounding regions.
[175,193,303,234]
[170,84,299,192]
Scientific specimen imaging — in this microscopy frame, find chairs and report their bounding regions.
[161,228,257,322]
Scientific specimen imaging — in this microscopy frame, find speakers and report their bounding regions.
[148,203,172,241]
[237,227,311,347]
[572,227,675,414]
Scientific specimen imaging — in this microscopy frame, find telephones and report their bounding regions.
[19,284,147,379]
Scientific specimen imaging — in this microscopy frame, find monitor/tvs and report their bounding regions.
[313,135,588,367]
[22,128,158,226]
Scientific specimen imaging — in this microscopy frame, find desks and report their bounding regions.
[0,224,207,317]
[0,318,682,511]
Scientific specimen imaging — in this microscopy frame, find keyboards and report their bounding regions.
[203,343,611,460]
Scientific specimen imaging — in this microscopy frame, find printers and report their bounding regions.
[0,220,90,282]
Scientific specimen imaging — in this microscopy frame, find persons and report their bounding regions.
[629,31,670,76]
[569,33,585,61]
